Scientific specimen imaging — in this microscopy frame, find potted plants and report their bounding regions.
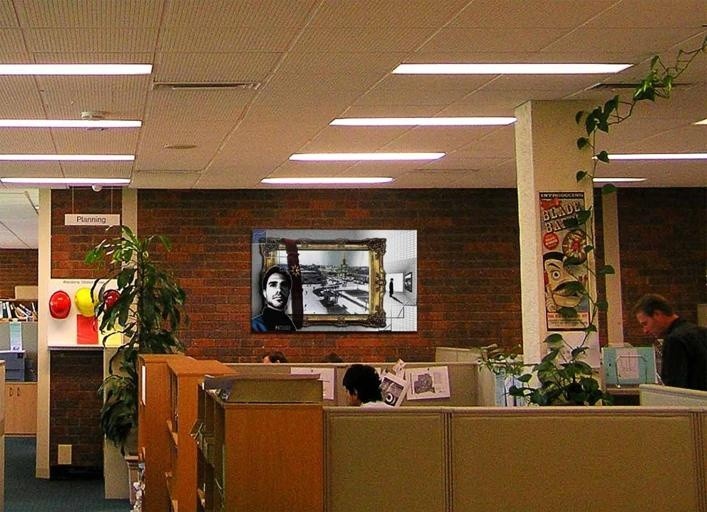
[87,225,188,507]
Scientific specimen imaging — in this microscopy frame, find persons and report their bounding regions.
[259,350,288,363]
[628,291,706,391]
[252,264,297,331]
[340,363,392,408]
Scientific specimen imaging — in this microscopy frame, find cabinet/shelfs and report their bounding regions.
[135,359,319,512]
[0,298,38,353]
[1,384,38,436]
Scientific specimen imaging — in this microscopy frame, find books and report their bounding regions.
[0,300,37,323]
[602,346,660,386]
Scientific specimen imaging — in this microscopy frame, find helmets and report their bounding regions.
[76,288,97,317]
[102,289,120,312]
[49,291,71,320]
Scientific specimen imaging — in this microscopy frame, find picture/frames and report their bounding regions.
[258,236,387,329]
